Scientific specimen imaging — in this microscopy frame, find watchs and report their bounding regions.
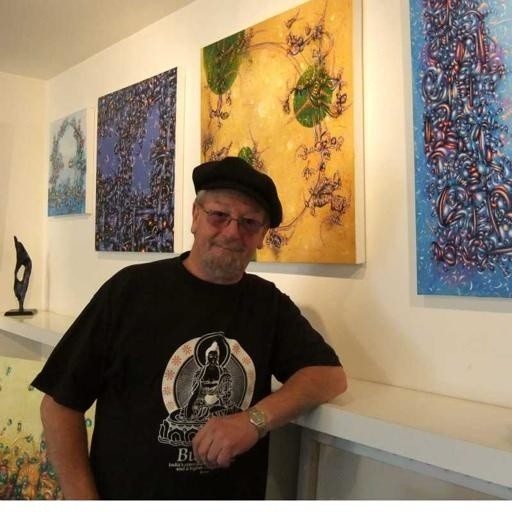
[242,405,267,441]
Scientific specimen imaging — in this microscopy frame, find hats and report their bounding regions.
[192,156,282,229]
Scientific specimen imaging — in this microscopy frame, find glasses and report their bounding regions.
[196,200,264,231]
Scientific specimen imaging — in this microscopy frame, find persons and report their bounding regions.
[34,156,346,500]
